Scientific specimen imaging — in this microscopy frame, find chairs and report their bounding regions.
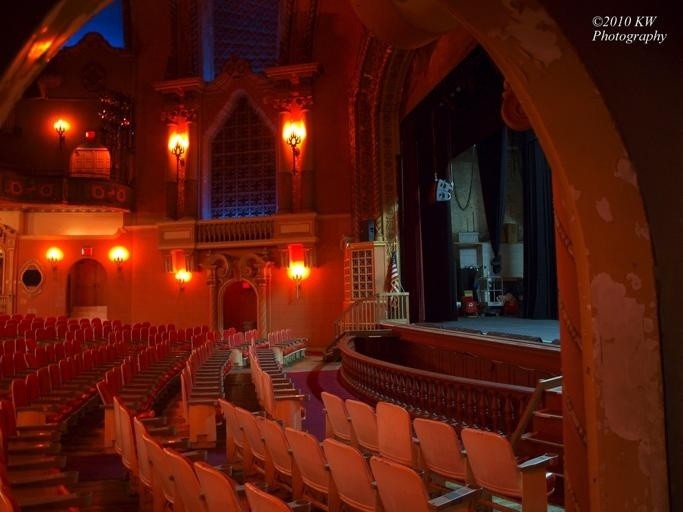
[0,307,558,511]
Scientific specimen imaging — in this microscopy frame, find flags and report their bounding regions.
[389,252,401,292]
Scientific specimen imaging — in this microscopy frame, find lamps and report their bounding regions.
[50,113,71,153]
[163,121,189,184]
[286,244,308,303]
[280,114,312,173]
[43,245,192,298]
